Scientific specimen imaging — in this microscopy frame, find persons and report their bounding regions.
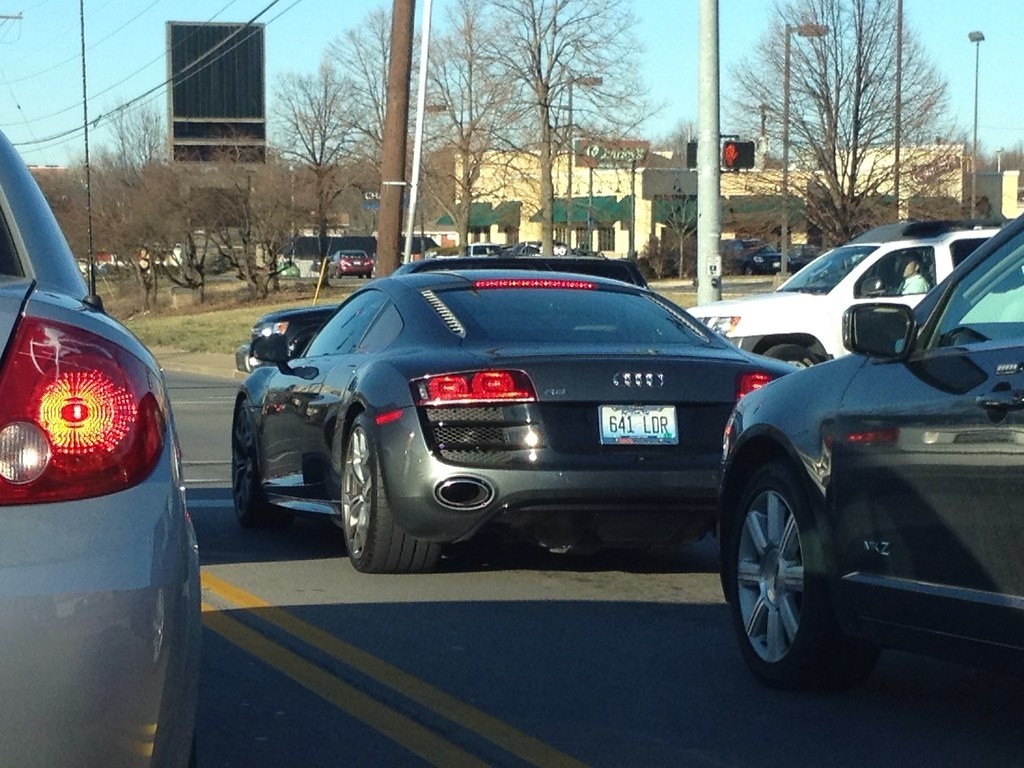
[900,260,930,294]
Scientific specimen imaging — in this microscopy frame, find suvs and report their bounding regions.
[687,220,1018,370]
[466,243,503,257]
[233,255,650,374]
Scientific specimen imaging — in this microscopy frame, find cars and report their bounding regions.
[717,231,825,275]
[1,123,204,767]
[332,249,375,279]
[278,262,301,276]
[501,240,572,255]
[229,268,820,572]
[715,209,1023,692]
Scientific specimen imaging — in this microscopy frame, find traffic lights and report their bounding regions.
[685,133,756,173]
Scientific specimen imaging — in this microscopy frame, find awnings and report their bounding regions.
[654,192,807,229]
[435,197,523,231]
[528,194,635,227]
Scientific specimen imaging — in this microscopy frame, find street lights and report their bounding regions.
[776,20,830,282]
[967,29,986,220]
